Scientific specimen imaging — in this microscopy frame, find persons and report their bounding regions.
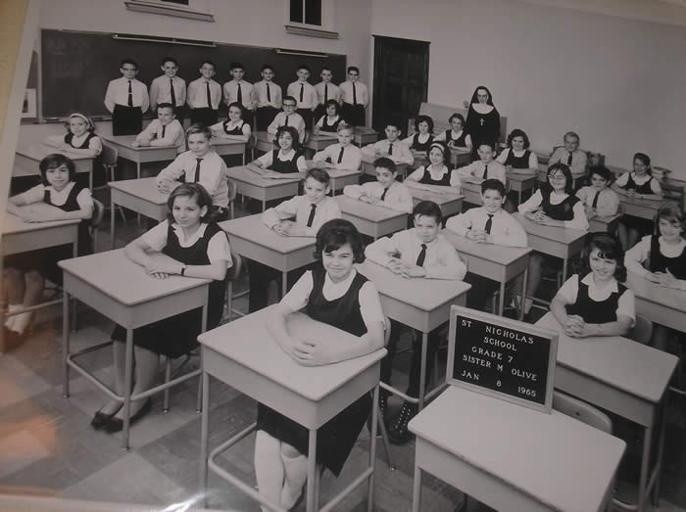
[103,57,149,137]
[186,60,223,125]
[156,121,229,224]
[252,218,388,511]
[207,102,252,143]
[284,64,318,126]
[464,84,499,150]
[250,64,281,132]
[363,201,467,445]
[309,99,346,137]
[622,199,686,356]
[312,67,344,121]
[149,57,187,124]
[89,181,233,432]
[40,111,106,190]
[0,154,95,353]
[265,95,306,147]
[131,103,187,156]
[339,66,369,126]
[222,61,253,126]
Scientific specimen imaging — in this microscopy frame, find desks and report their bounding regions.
[2,103,684,510]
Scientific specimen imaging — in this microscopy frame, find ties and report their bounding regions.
[195,157,203,183]
[337,146,345,163]
[285,116,289,126]
[380,188,388,200]
[388,143,393,155]
[567,151,572,165]
[484,213,494,235]
[592,191,600,208]
[162,125,166,137]
[482,164,490,182]
[307,203,317,227]
[416,243,427,266]
[127,79,361,107]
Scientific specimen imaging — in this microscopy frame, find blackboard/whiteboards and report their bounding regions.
[445,304,559,414]
[36,27,347,123]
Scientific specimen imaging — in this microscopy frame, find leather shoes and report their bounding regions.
[0,314,29,353]
[91,392,122,428]
[107,395,152,432]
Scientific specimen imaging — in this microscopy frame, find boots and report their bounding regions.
[387,397,418,445]
[366,389,389,431]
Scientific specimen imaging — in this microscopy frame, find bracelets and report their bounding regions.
[180,264,186,276]
